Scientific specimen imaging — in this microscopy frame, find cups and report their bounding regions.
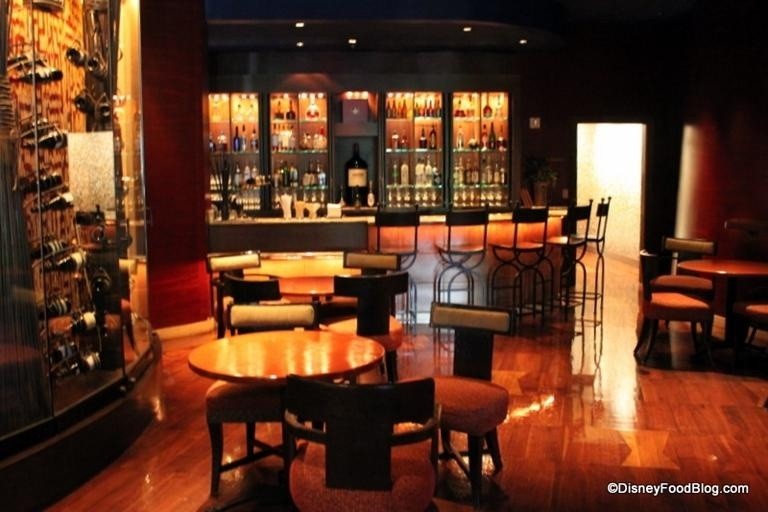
[533,182,547,205]
[280,194,320,219]
[207,209,216,224]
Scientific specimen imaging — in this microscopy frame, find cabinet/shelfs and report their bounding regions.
[208,90,511,217]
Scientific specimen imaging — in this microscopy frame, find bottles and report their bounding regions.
[8,47,124,380]
[385,94,507,208]
[367,180,376,207]
[208,97,327,189]
[342,143,369,207]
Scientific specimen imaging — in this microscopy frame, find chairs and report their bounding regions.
[632,216,768,367]
[184,194,613,512]
